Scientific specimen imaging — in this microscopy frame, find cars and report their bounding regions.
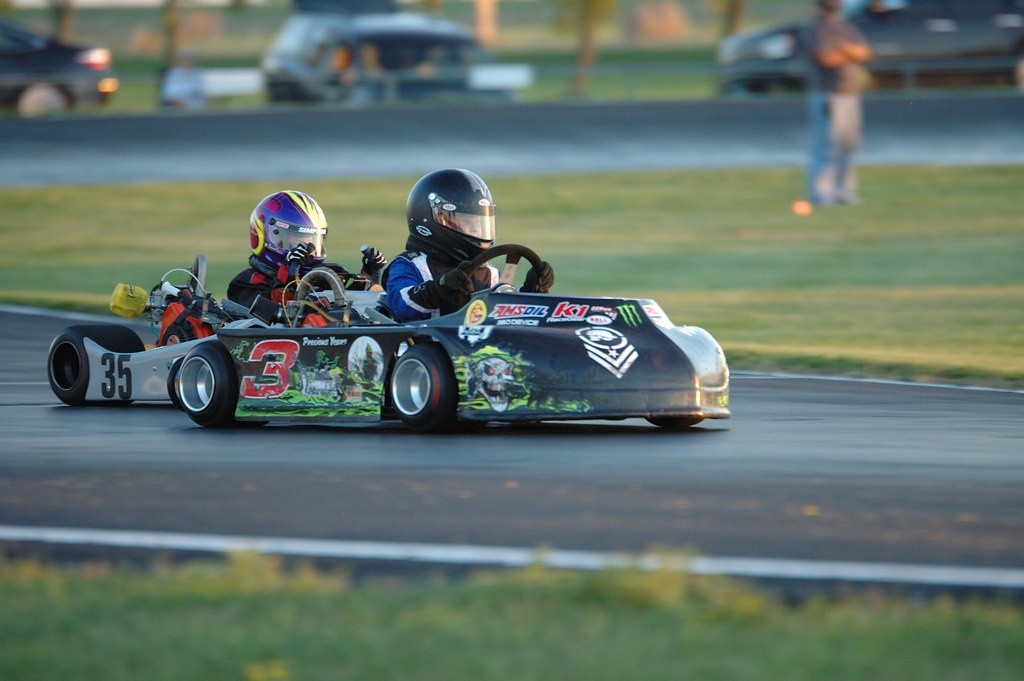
[719,0,1024,79]
[0,24,115,118]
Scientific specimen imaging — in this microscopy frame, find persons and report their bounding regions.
[799,0,872,212]
[227,190,387,309]
[381,169,554,323]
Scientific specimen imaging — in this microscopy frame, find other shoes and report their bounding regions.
[811,191,866,208]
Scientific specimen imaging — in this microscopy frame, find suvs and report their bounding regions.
[263,0,513,106]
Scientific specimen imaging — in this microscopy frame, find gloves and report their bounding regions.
[361,247,387,275]
[520,261,554,294]
[434,259,475,305]
[286,242,309,264]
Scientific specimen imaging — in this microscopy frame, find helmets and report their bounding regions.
[249,190,330,278]
[405,168,496,261]
